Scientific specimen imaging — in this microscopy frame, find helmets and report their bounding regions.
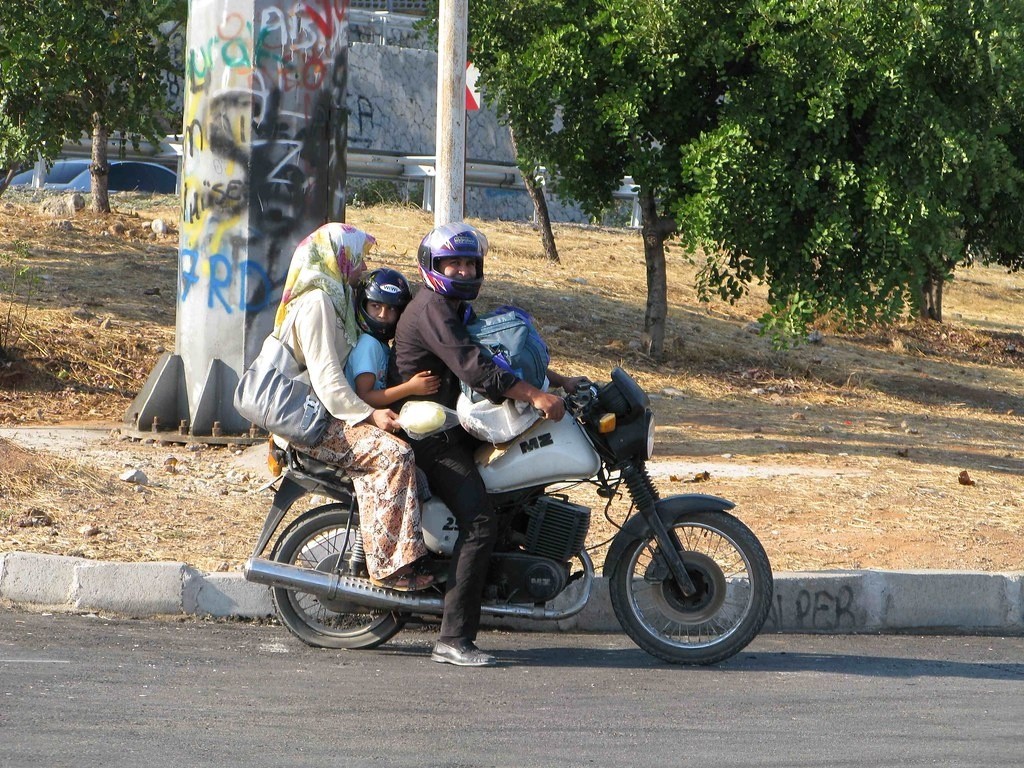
[418,222,488,300]
[354,267,412,341]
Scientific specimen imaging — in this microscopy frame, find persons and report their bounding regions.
[345,267,443,527]
[388,223,594,667]
[267,222,435,592]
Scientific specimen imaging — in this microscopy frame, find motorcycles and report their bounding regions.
[243,366,776,664]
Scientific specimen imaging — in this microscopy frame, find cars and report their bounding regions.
[9,157,176,197]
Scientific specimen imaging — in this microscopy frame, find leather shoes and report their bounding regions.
[430,640,496,667]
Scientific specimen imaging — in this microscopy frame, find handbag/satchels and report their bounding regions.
[460,304,551,404]
[233,290,333,448]
[456,373,549,444]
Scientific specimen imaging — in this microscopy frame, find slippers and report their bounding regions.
[369,564,433,591]
